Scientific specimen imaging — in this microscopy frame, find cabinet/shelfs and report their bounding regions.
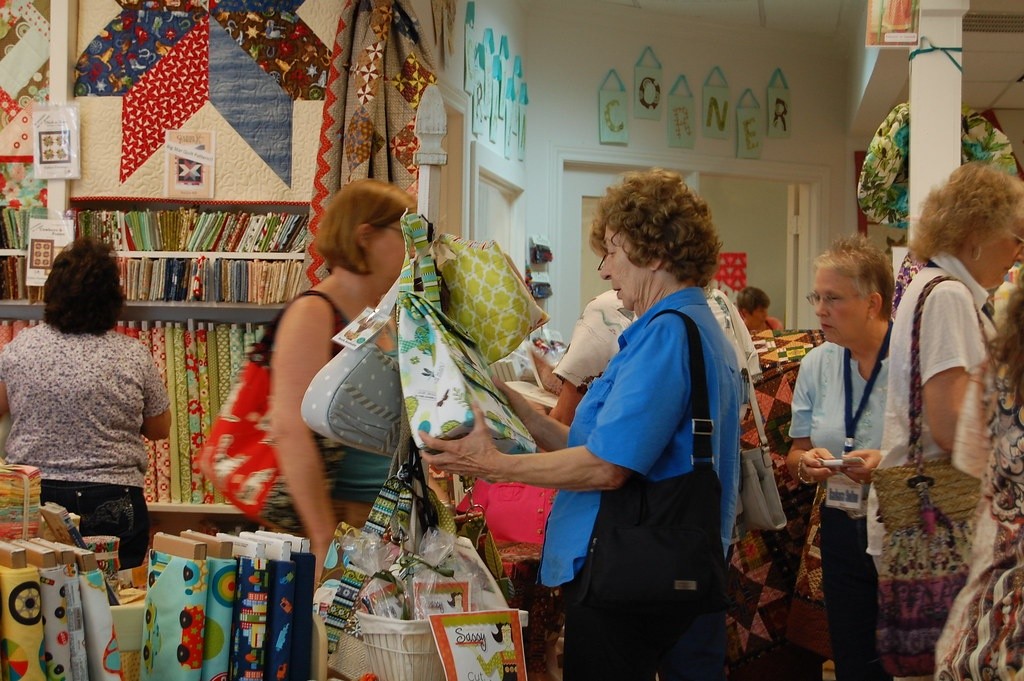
[0,249,306,515]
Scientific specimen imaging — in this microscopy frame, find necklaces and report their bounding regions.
[851,349,875,374]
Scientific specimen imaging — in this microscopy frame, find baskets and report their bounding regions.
[355,608,445,681]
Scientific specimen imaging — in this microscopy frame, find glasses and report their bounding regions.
[806,289,873,307]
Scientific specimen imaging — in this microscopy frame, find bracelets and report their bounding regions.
[798,451,817,484]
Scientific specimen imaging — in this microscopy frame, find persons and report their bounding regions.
[416,170,762,681]
[866,166,1024,681]
[0,237,172,570]
[790,232,893,681]
[735,287,783,331]
[268,179,419,594]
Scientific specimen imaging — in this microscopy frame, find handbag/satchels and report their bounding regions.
[870,456,981,677]
[566,309,732,620]
[200,291,351,538]
[299,207,557,681]
[738,446,787,531]
[859,102,1018,227]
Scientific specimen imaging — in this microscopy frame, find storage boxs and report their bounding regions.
[0,530,528,681]
